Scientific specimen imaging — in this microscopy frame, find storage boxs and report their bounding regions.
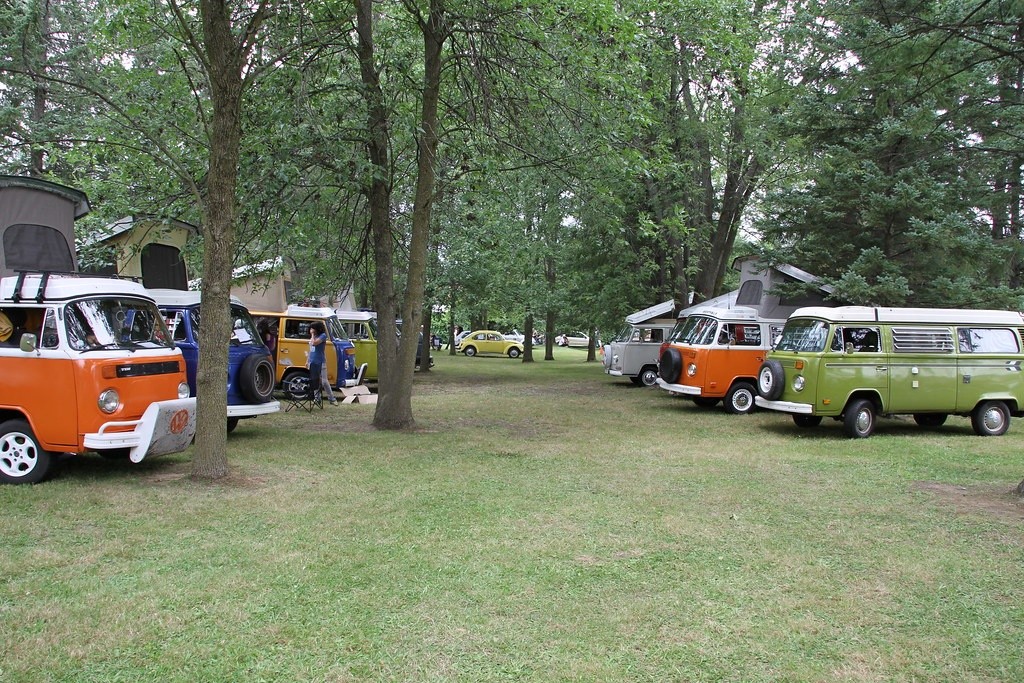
[339,384,378,404]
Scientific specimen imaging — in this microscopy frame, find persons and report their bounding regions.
[490,336,494,341]
[305,321,339,406]
[455,325,463,336]
[532,328,537,341]
[257,321,276,365]
[85,328,100,346]
[431,335,443,350]
[445,326,453,349]
[558,336,569,347]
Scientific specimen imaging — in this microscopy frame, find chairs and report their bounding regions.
[282,363,323,413]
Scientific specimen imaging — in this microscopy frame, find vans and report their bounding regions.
[654,307,788,417]
[121,288,281,432]
[247,311,356,401]
[336,313,434,380]
[753,305,1024,438]
[0,269,197,486]
[600,300,678,388]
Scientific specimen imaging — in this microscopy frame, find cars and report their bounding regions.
[501,330,535,346]
[454,331,485,348]
[458,331,524,358]
[556,331,601,348]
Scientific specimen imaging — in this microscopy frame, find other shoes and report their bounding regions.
[330,400,338,406]
[311,399,322,404]
